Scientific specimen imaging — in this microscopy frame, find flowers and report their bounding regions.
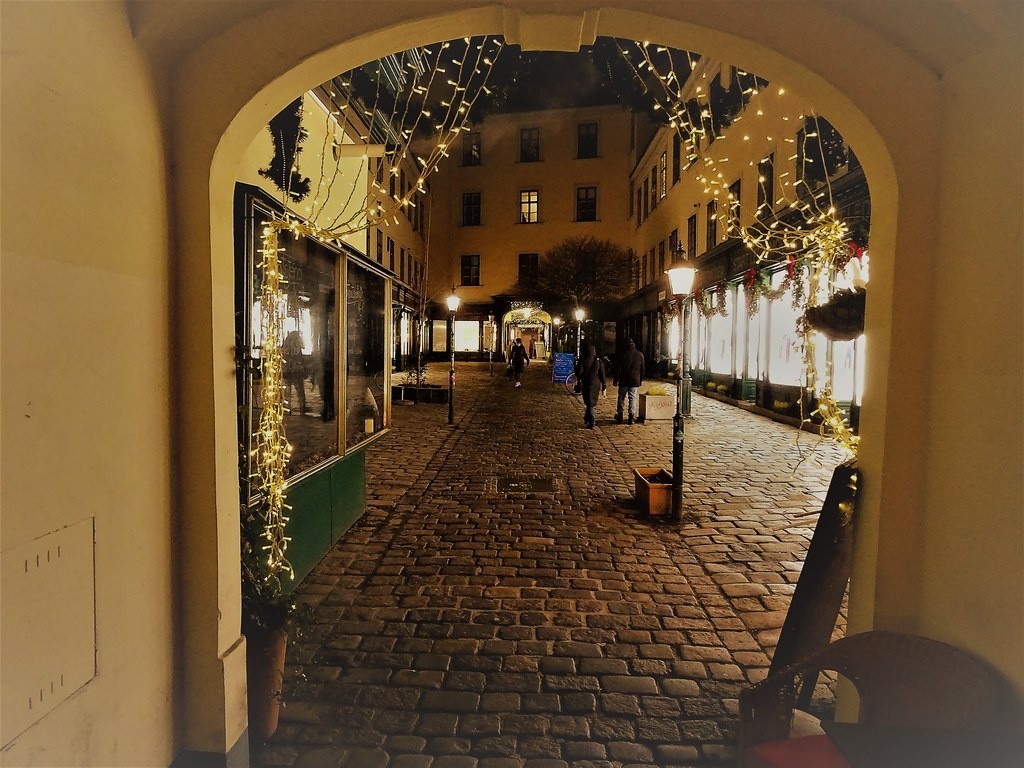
[774,399,788,408]
[659,225,869,324]
[717,385,727,391]
[826,257,867,301]
[706,382,716,388]
[646,387,668,396]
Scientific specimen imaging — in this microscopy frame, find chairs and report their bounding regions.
[735,630,1000,768]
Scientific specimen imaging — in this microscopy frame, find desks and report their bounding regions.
[819,721,1023,768]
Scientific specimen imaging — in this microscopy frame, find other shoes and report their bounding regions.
[615,411,623,423]
[583,416,596,430]
[628,413,634,424]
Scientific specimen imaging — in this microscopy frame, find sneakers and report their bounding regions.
[515,382,522,388]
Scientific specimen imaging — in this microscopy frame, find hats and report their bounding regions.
[623,338,635,345]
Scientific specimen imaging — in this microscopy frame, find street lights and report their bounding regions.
[574,306,586,359]
[664,239,700,526]
[447,285,462,422]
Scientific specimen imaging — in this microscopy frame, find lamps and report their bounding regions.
[332,138,402,161]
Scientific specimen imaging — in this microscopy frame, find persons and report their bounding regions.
[280,331,334,421]
[528,335,537,360]
[612,338,645,425]
[576,344,606,429]
[508,338,530,387]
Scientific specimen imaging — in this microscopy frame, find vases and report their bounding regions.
[633,468,673,514]
[805,291,866,341]
[639,391,675,419]
[668,376,673,379]
[707,387,713,391]
[776,408,788,414]
[667,373,674,376]
[718,390,725,395]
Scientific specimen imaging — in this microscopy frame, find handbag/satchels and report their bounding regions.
[504,364,513,377]
